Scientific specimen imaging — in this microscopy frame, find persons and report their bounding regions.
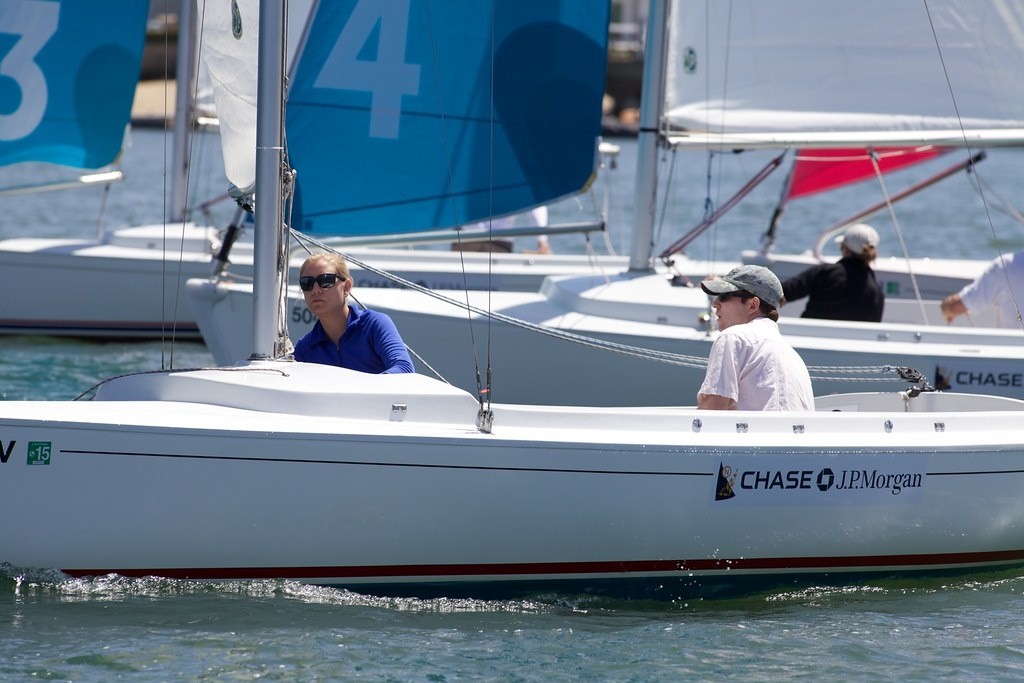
[452,205,553,254]
[697,265,815,411]
[293,254,415,374]
[697,225,884,322]
[941,250,1024,329]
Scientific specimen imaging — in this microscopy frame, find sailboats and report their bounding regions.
[1,1,1024,606]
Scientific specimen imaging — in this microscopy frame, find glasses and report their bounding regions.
[299,272,346,291]
[717,290,753,302]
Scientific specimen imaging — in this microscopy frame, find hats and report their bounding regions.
[701,265,784,312]
[834,223,879,256]
